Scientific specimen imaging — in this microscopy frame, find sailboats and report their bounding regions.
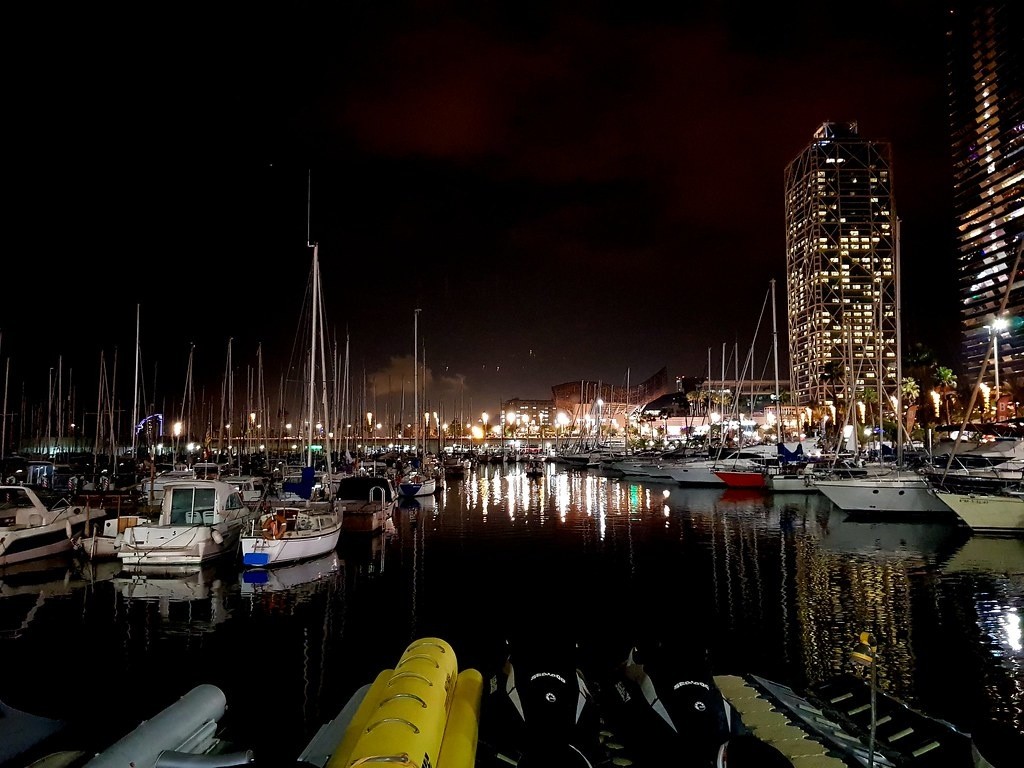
[0,218,1024,578]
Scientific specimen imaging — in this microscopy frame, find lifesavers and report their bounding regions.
[259,514,286,541]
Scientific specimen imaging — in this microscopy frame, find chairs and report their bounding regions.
[203,511,213,525]
[186,512,202,524]
